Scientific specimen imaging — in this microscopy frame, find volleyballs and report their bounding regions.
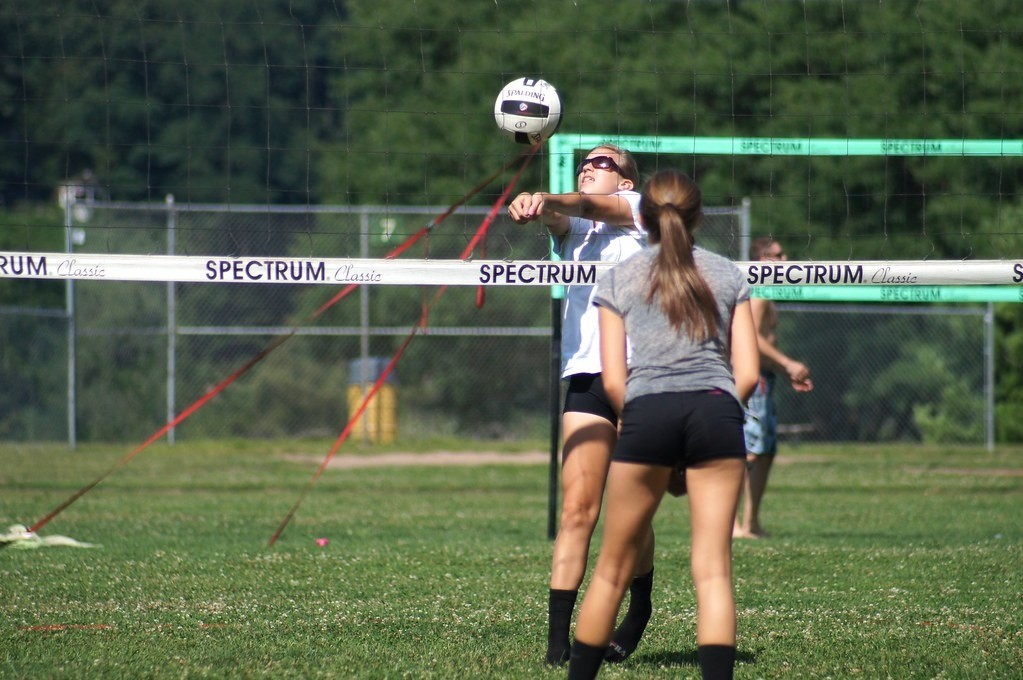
[494,75,564,149]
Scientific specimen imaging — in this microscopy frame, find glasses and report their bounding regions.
[575,156,628,180]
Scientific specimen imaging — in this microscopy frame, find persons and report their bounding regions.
[731,233,814,539]
[504,142,656,668]
[564,171,761,680]
